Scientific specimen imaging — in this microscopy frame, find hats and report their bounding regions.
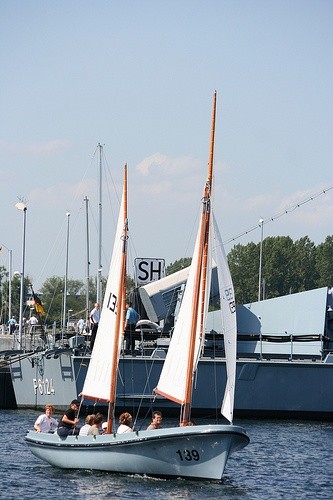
[72,400,81,405]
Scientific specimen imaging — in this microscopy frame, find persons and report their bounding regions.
[33,404,59,433]
[89,303,100,354]
[28,313,38,332]
[80,414,95,436]
[89,413,103,436]
[8,316,17,335]
[146,412,163,431]
[73,316,92,335]
[57,399,80,436]
[122,302,141,357]
[117,412,133,434]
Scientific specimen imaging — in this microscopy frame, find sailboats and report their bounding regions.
[23,86,251,483]
